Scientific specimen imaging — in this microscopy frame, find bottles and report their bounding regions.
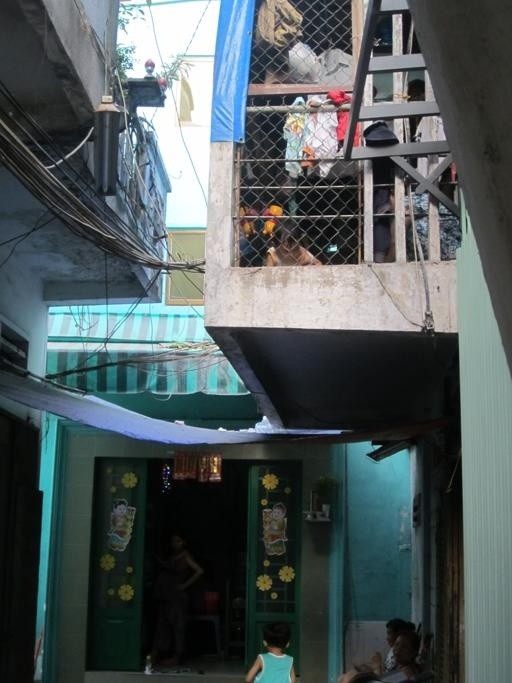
[143,655,152,675]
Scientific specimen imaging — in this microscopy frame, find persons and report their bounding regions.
[142,533,205,664]
[347,628,427,683]
[336,617,417,682]
[264,220,323,266]
[369,76,463,263]
[244,620,297,683]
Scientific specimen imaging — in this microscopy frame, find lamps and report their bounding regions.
[173,450,223,482]
